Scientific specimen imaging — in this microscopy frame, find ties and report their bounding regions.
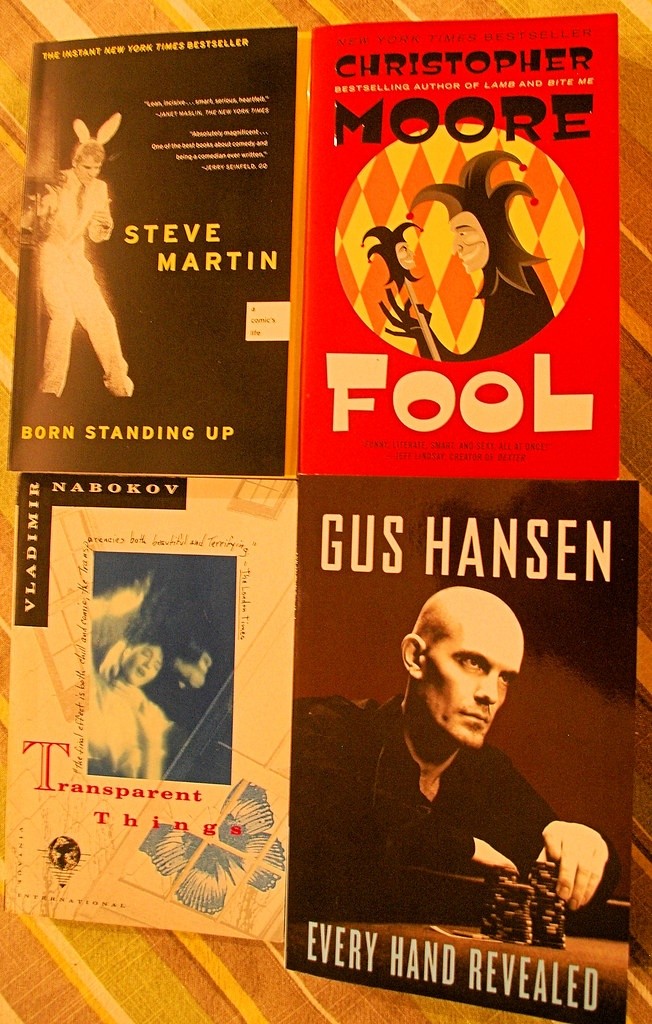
[77,186,86,216]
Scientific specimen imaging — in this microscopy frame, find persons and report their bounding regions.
[85,635,229,782]
[34,113,135,397]
[288,580,613,926]
